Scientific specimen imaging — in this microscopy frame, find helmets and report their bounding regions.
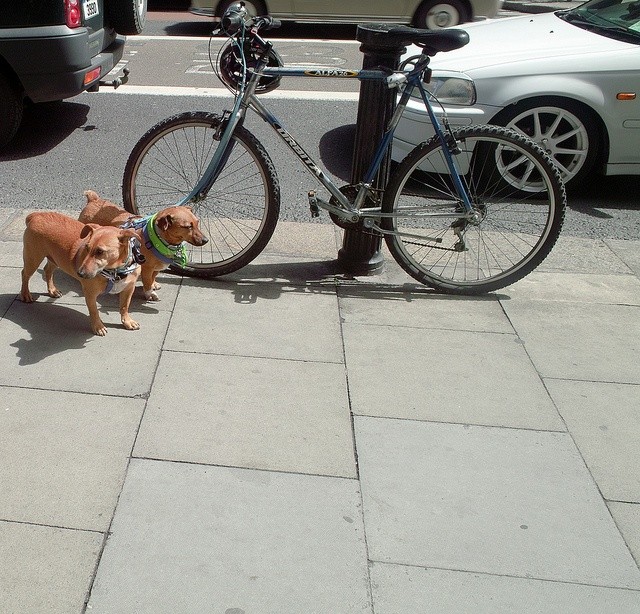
[220,40,285,93]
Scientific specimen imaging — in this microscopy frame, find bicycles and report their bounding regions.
[122,1,565,296]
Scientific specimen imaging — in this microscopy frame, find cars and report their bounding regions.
[389,2,640,200]
[187,0,503,35]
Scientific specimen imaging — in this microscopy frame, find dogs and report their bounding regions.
[77,189,209,301]
[20,211,142,337]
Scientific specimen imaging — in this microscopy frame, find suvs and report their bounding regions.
[1,1,147,147]
